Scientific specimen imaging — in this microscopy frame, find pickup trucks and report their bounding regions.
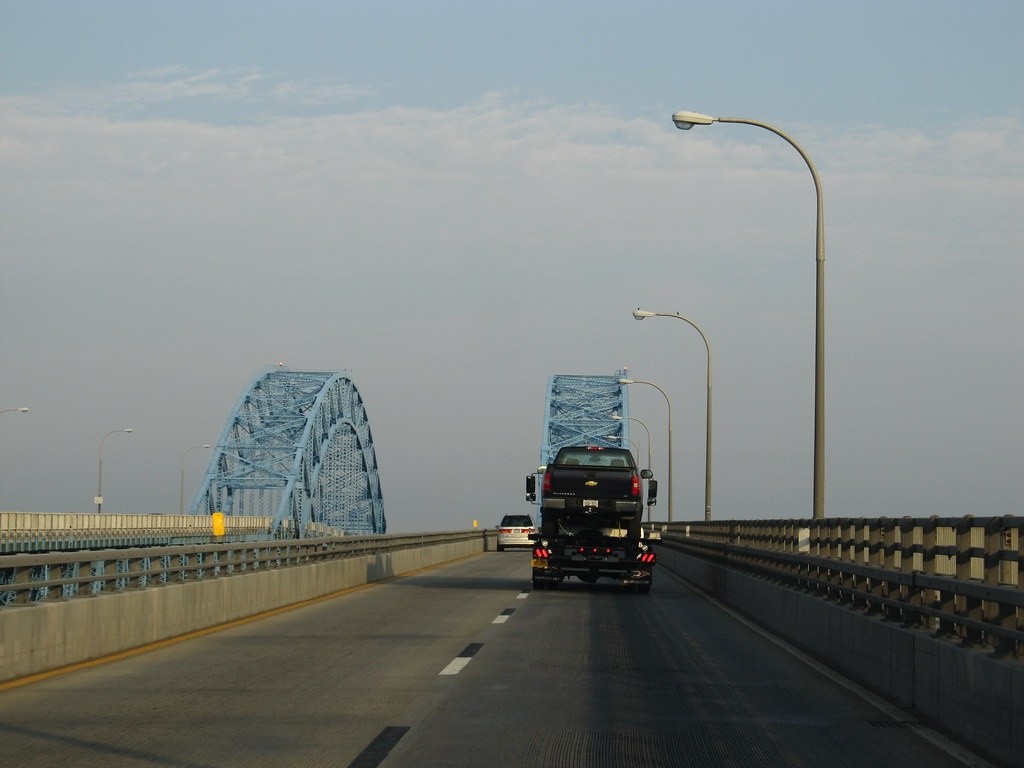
[538,445,653,535]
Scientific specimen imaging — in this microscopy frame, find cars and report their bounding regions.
[494,514,536,551]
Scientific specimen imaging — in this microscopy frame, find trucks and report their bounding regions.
[526,444,658,594]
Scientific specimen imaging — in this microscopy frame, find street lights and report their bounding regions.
[0,407,29,413]
[671,109,827,519]
[179,443,210,515]
[608,377,675,522]
[633,307,716,524]
[96,428,132,513]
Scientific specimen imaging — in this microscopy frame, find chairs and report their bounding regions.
[523,521,531,527]
[565,458,580,465]
[610,459,626,467]
